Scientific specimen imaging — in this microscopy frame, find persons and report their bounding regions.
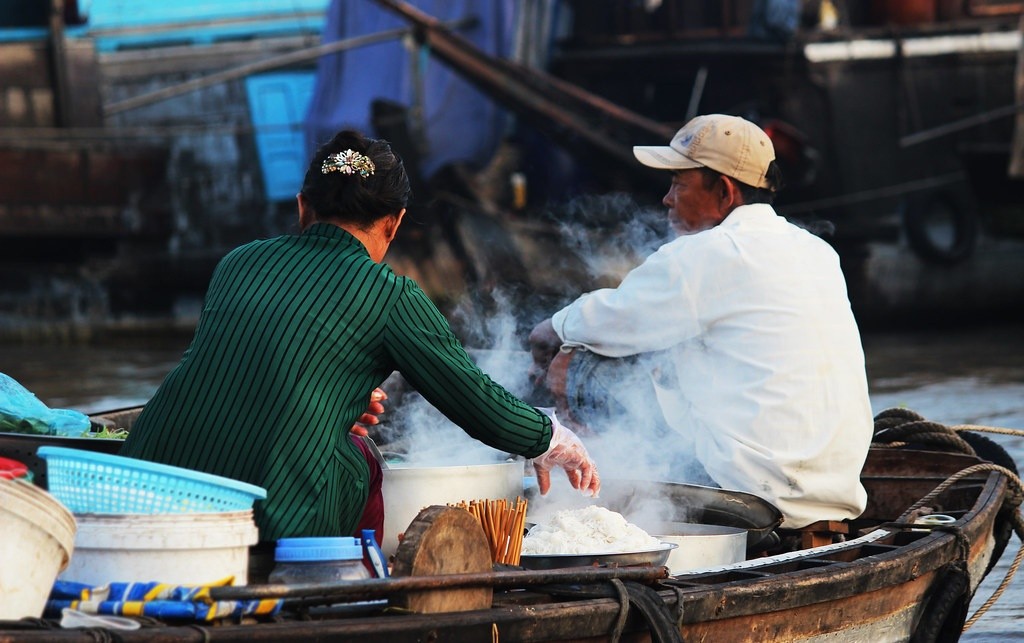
[527,115,877,532]
[123,126,601,580]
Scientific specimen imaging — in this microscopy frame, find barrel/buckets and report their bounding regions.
[55,506,259,588]
[377,462,525,561]
[0,473,78,623]
[634,518,750,576]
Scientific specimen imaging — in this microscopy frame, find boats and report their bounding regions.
[370,2,1024,342]
[0,402,1024,643]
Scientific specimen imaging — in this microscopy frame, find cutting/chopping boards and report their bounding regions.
[384,505,500,613]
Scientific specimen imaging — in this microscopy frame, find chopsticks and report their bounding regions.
[441,495,532,568]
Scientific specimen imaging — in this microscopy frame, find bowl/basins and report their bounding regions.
[518,544,676,570]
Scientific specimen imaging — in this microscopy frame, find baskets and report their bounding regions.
[36,445,266,515]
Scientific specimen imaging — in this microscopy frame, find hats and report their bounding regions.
[632,115,775,190]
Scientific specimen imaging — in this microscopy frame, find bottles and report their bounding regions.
[268,532,373,619]
